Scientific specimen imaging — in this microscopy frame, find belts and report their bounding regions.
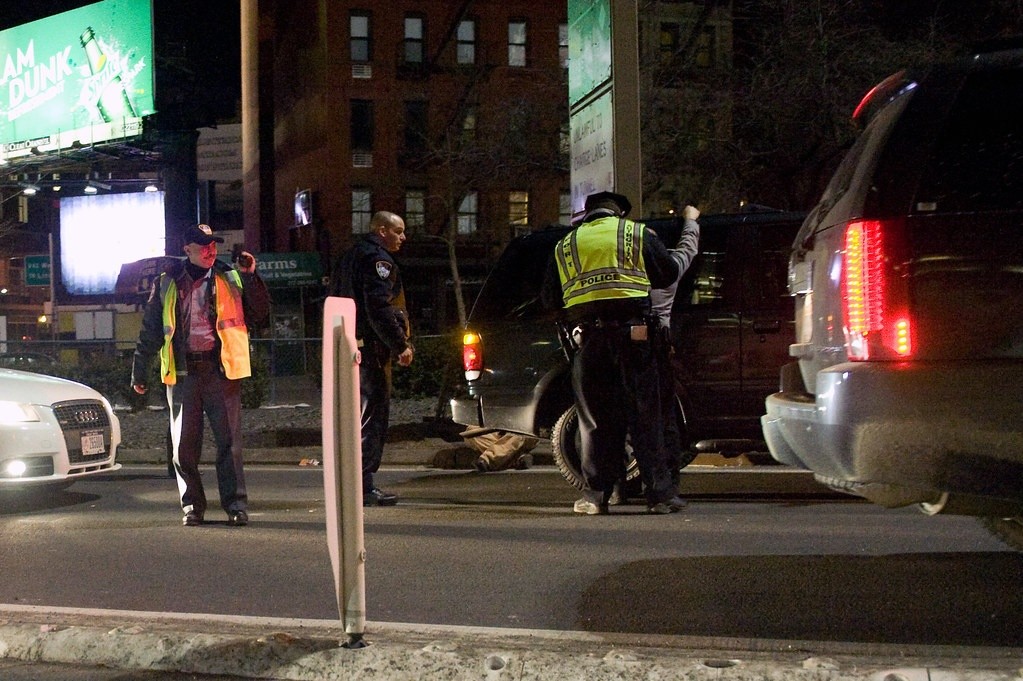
[185,350,219,363]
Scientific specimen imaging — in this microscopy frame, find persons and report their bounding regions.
[130,224,271,526]
[459,421,541,471]
[324,211,413,505]
[554,189,700,515]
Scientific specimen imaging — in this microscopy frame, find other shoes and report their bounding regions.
[608,485,628,505]
[573,498,609,514]
[648,496,687,513]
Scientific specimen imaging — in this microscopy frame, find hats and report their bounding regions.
[584,191,633,218]
[182,223,225,246]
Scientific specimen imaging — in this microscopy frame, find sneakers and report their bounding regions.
[229,510,248,526]
[182,510,204,525]
[364,487,398,506]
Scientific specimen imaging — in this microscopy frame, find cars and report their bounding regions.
[0,369,122,491]
[448,197,794,501]
[758,48,1023,554]
